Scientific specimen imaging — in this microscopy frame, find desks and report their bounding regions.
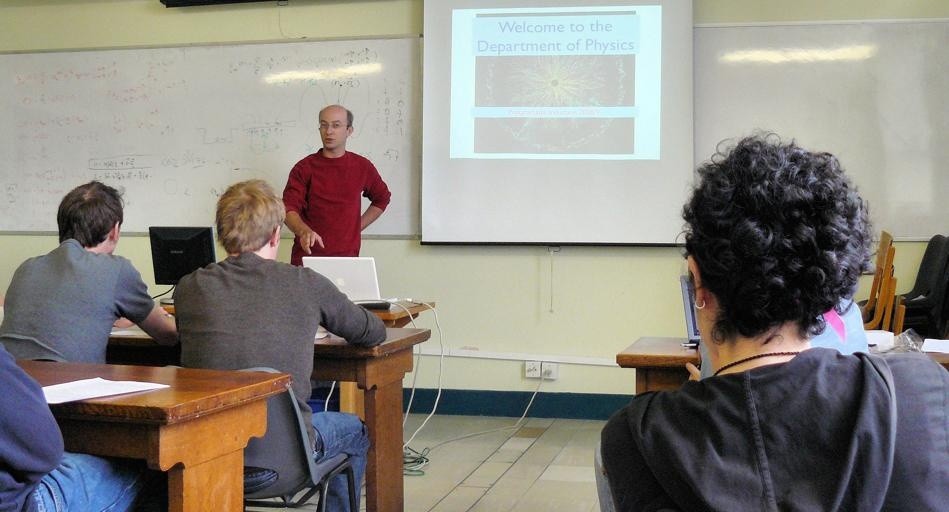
[156,300,437,425]
[108,324,430,511]
[615,336,949,397]
[14,358,298,512]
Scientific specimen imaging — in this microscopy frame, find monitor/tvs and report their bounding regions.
[149,226,217,304]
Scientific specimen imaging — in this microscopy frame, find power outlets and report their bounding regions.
[541,361,557,380]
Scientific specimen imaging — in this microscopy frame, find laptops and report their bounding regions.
[680,275,701,350]
[302,257,398,304]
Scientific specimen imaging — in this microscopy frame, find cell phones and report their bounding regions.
[357,302,390,310]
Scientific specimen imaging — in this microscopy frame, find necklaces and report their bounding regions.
[706,348,812,376]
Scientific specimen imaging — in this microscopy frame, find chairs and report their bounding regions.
[854,230,949,342]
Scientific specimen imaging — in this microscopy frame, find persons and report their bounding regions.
[680,297,868,385]
[281,108,390,273]
[172,179,386,512]
[595,133,949,511]
[0,179,180,363]
[0,335,162,512]
